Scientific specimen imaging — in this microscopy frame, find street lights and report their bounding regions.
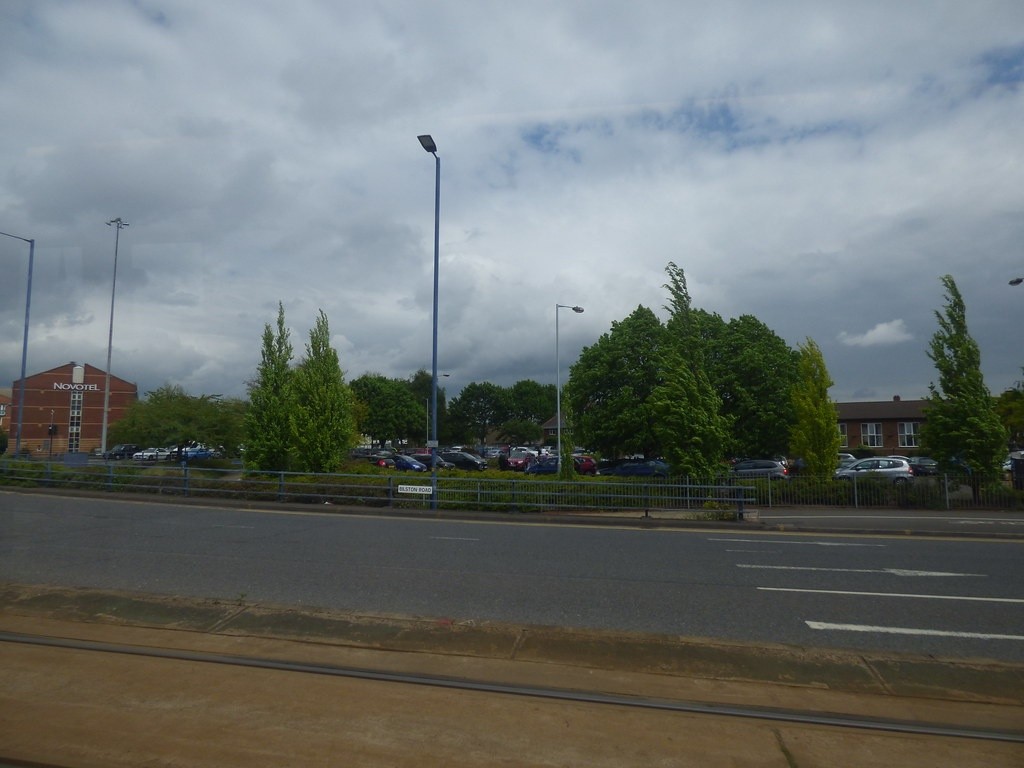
[553,301,585,482]
[418,136,444,482]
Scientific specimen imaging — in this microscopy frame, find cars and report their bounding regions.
[102,440,1023,479]
